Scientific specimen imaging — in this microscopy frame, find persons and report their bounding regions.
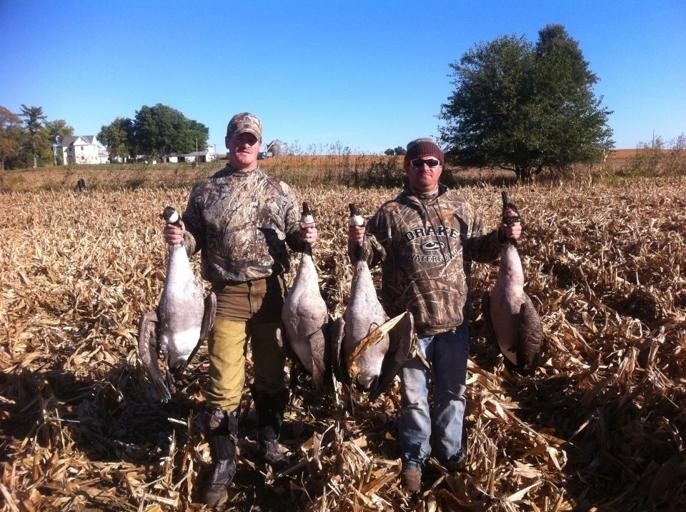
[348,138,523,493]
[162,112,318,507]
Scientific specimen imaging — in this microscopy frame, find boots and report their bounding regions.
[198,405,241,508]
[249,382,290,472]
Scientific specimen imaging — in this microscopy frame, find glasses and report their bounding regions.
[410,158,441,169]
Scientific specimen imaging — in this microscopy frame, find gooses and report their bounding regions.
[136,205,217,405]
[331,203,416,416]
[282,200,333,395]
[481,190,543,378]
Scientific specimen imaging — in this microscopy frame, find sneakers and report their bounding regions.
[402,468,422,491]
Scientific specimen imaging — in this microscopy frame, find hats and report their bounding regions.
[226,112,262,140]
[404,138,444,172]
